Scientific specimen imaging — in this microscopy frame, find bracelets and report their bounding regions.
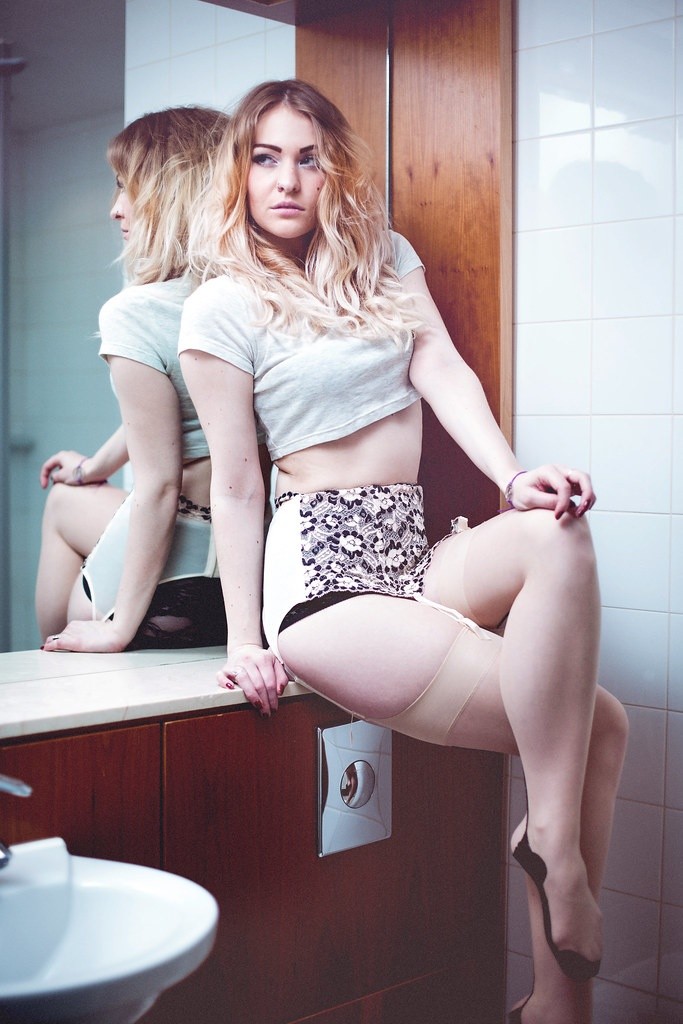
[74,456,91,485]
[498,471,526,512]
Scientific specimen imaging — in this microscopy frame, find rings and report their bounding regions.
[53,635,58,640]
[234,670,240,677]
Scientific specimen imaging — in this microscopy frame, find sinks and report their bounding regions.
[0,837,220,1024]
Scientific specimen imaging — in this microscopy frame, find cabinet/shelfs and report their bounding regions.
[0,698,510,1023]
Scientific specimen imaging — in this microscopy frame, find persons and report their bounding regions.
[175,79,632,1024]
[35,105,233,655]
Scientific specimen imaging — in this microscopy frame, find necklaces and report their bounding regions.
[250,224,306,264]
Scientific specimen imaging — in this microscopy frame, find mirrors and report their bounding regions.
[1,1,393,637]
